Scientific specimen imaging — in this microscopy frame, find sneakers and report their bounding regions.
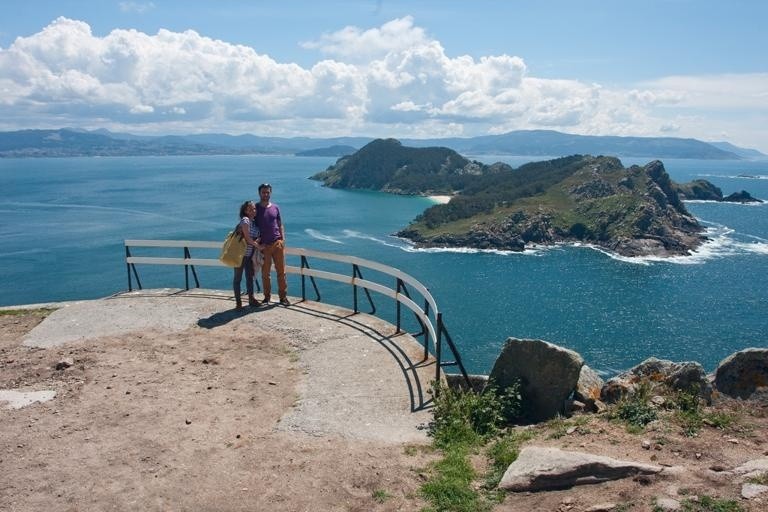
[280,298,290,305]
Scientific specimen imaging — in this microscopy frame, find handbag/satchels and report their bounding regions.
[220,219,247,268]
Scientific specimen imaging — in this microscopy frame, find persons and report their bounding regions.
[219,200,262,312]
[251,181,290,306]
